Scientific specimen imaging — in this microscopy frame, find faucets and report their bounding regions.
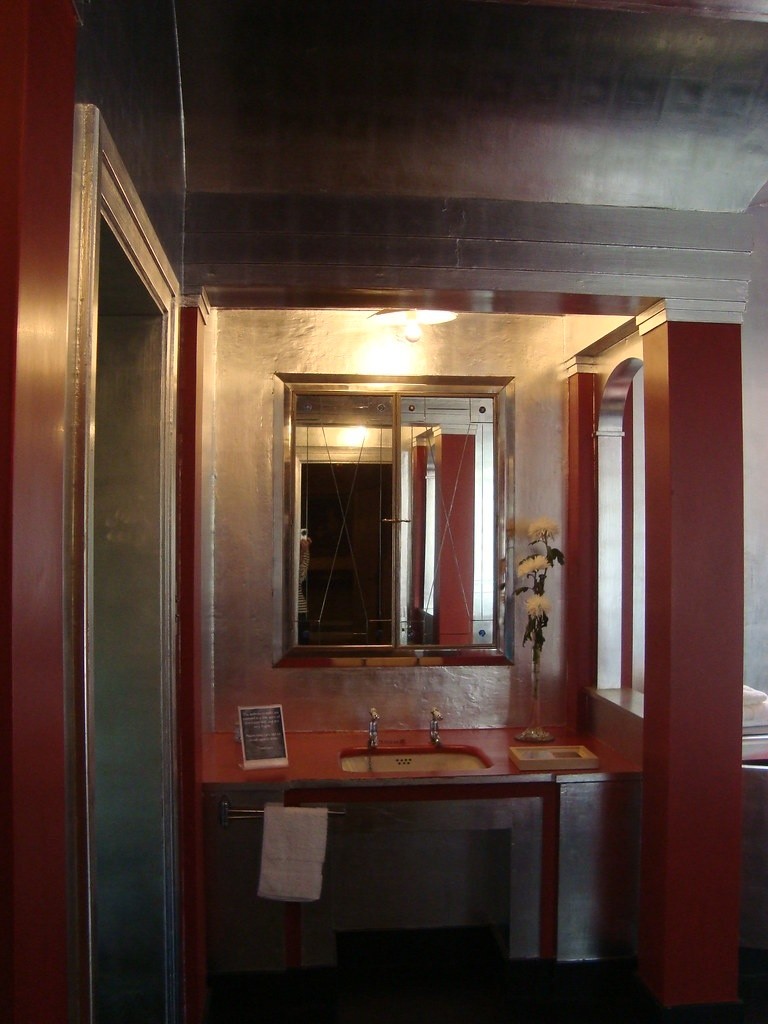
[428,707,445,750]
[367,707,381,749]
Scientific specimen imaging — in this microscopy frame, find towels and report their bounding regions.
[256,804,329,904]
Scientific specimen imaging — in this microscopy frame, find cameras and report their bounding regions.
[300,529,307,541]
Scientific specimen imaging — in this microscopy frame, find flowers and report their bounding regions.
[512,517,564,698]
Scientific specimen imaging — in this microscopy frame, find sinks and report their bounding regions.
[338,744,495,774]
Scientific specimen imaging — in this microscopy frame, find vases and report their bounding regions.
[516,647,556,743]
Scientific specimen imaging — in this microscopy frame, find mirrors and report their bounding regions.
[295,394,498,648]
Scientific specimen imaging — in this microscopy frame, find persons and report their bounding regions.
[298,537,313,645]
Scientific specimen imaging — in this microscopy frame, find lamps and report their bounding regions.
[367,310,458,343]
